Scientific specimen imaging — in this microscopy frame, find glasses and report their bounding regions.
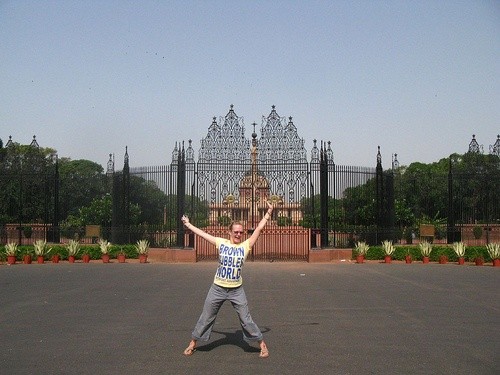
[234,231,243,234]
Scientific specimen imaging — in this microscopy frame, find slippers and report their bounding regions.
[183,346,196,356]
[260,347,269,357]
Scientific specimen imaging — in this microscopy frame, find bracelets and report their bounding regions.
[264,216,268,219]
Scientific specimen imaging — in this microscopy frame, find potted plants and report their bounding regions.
[31,238,53,264]
[485,241,500,266]
[418,240,434,263]
[52,251,59,264]
[404,247,413,264]
[98,238,111,263]
[133,239,150,263]
[118,248,126,262]
[22,248,34,264]
[438,246,448,264]
[82,249,89,263]
[2,240,21,265]
[452,240,467,265]
[23,225,33,238]
[474,249,483,266]
[64,238,82,263]
[354,240,371,263]
[381,240,396,263]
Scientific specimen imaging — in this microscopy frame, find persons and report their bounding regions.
[181,201,274,358]
